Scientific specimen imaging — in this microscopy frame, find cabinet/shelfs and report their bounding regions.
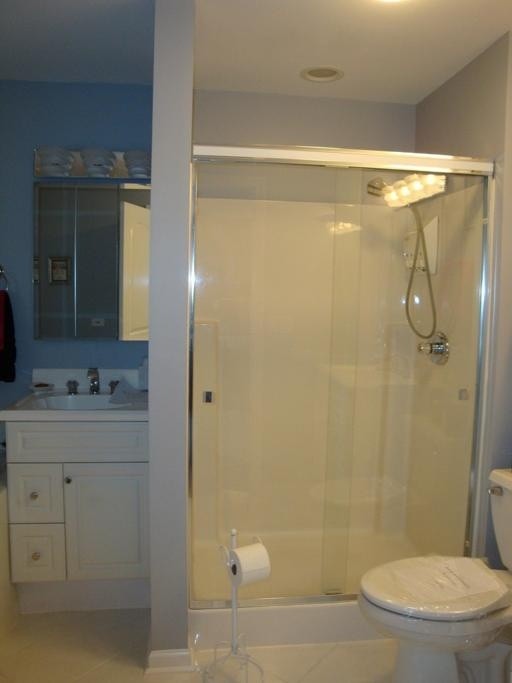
[5,418,151,584]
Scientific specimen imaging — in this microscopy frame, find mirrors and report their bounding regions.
[30,181,149,342]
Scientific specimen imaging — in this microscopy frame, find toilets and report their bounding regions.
[358,470,512,683]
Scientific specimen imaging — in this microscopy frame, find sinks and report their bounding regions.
[31,395,127,409]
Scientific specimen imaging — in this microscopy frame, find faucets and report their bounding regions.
[87,367,99,394]
